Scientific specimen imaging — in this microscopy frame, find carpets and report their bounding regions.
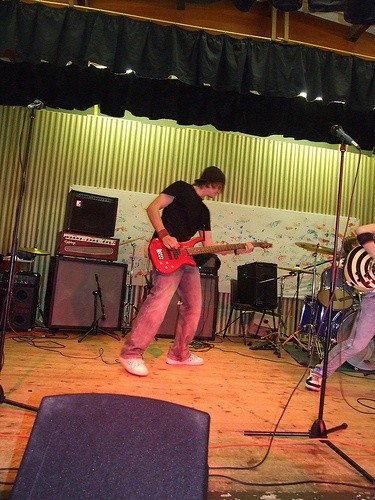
[282,345,375,373]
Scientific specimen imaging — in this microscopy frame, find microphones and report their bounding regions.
[330,125,362,151]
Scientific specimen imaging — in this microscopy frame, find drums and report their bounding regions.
[295,293,318,337]
[316,265,355,311]
[329,307,375,371]
[317,305,356,342]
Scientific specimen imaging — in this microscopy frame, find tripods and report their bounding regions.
[249,151,375,486]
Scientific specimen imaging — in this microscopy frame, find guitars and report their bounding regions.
[344,245,375,292]
[146,236,274,275]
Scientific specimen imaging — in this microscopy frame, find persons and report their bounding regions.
[303,224,375,389]
[120,167,254,376]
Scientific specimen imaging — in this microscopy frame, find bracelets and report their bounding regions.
[158,229,169,239]
[234,249,239,255]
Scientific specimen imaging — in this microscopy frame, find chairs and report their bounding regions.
[223,279,280,344]
[8,394,210,500]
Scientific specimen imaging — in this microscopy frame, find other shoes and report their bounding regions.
[119,358,149,376]
[167,355,203,365]
[305,376,320,391]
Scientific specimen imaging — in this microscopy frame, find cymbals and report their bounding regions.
[277,266,319,276]
[341,235,360,257]
[18,247,52,257]
[295,240,342,257]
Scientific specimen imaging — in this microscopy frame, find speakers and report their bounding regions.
[249,312,280,339]
[63,189,118,237]
[10,392,210,500]
[0,270,40,332]
[44,256,128,330]
[150,272,219,341]
[236,262,277,308]
